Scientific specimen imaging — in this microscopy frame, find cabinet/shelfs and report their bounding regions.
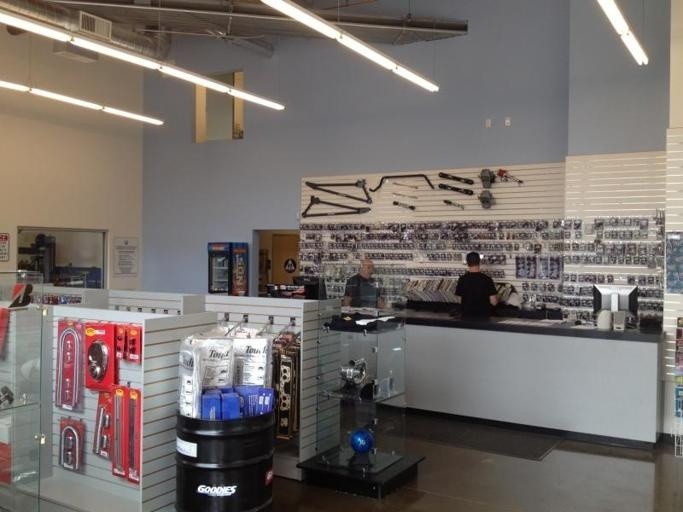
[331,312,662,451]
[0,263,426,512]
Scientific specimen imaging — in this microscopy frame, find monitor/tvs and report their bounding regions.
[592,283,638,327]
[292,276,327,299]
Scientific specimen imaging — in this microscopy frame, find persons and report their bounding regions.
[343,258,386,308]
[454,252,502,312]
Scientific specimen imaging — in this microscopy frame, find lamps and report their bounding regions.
[597,1,650,68]
[1,80,165,127]
[261,1,441,95]
[1,9,286,112]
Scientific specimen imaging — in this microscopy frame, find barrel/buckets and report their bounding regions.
[176,411,274,512]
